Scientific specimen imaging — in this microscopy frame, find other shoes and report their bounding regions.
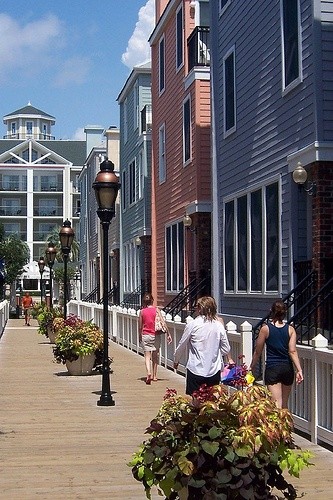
[24,323,29,326]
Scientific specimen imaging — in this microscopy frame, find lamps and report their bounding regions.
[110,248,119,260]
[293,161,318,198]
[135,235,145,250]
[184,214,198,234]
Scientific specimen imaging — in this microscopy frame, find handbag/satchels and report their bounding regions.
[220,353,243,386]
[155,307,167,335]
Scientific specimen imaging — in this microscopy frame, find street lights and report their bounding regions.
[92,156,120,407]
[44,239,59,309]
[37,257,46,305]
[58,218,76,322]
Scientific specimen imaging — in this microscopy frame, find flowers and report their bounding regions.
[47,313,114,368]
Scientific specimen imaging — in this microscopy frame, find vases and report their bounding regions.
[47,328,59,344]
[66,352,96,375]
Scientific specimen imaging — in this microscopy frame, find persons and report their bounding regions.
[22,292,33,326]
[173,297,235,396]
[249,301,303,409]
[139,293,172,385]
